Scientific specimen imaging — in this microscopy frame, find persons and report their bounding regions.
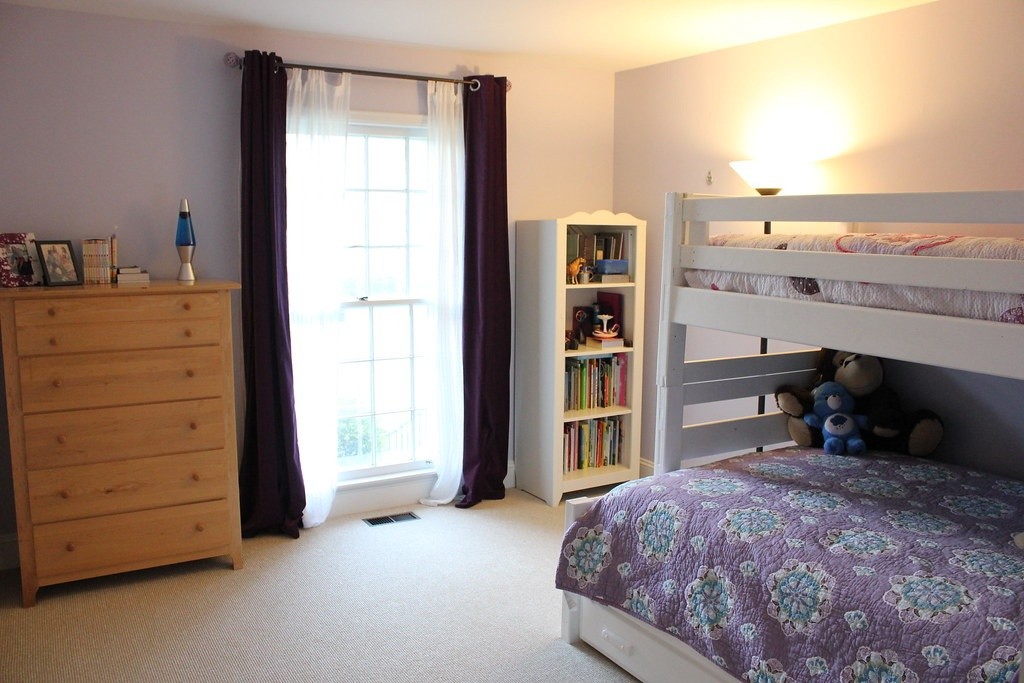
[10,247,27,274]
[48,246,75,279]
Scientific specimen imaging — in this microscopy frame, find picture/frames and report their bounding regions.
[0,232,40,288]
[34,238,80,286]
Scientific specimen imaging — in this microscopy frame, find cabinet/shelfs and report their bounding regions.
[512,209,647,506]
[0,277,244,607]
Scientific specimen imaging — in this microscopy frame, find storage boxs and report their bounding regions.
[598,259,628,274]
[600,274,630,284]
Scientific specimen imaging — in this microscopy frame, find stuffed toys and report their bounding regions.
[775,347,944,456]
[804,381,869,456]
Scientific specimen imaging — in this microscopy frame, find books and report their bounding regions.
[82,235,149,283]
[574,292,623,348]
[566,232,630,283]
[564,353,628,411]
[563,416,624,474]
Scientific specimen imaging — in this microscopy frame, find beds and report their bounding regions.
[555,185,1023,683]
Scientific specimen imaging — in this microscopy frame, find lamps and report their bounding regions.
[728,154,802,453]
